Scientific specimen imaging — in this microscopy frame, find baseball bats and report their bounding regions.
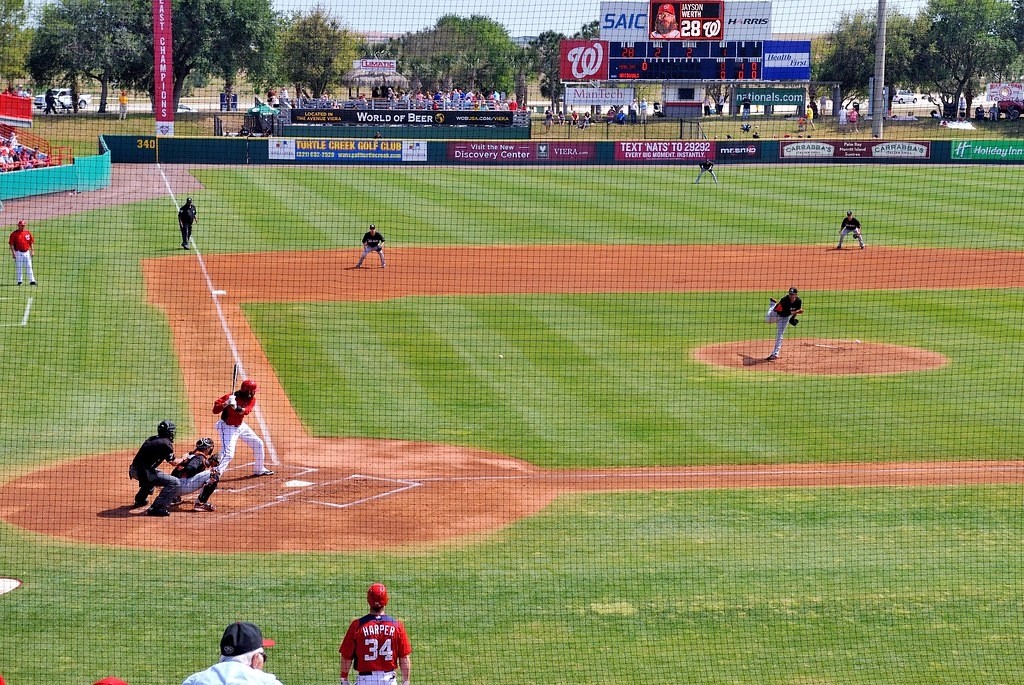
[231,364,238,407]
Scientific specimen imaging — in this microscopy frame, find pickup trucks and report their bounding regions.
[34,89,92,110]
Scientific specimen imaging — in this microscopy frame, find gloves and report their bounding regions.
[225,395,236,405]
[340,673,349,685]
[230,400,237,410]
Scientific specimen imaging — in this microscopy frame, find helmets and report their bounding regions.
[158,420,176,437]
[241,380,258,393]
[197,437,214,450]
[368,583,389,608]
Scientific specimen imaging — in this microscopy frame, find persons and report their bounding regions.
[182,622,283,685]
[704,93,859,139]
[694,158,718,184]
[375,132,382,138]
[43,89,57,114]
[225,125,272,137]
[959,97,985,122]
[545,98,648,133]
[212,381,274,476]
[170,438,221,512]
[355,224,386,268]
[128,421,189,517]
[119,90,128,120]
[338,583,413,685]
[836,211,865,249]
[0,132,46,172]
[267,87,528,128]
[989,103,999,122]
[0,82,33,98]
[178,198,198,250]
[9,221,37,286]
[650,4,681,38]
[766,287,804,362]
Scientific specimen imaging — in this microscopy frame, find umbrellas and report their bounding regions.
[248,103,280,123]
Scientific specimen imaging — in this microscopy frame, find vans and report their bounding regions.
[892,90,917,104]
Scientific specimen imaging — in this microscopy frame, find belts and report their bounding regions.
[226,425,238,427]
[359,670,394,676]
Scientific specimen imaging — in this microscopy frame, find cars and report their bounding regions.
[176,104,199,113]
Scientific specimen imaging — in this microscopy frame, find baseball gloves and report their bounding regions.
[789,317,798,326]
[853,234,859,239]
[375,244,382,251]
[208,454,220,467]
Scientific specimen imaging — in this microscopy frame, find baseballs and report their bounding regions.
[499,354,503,358]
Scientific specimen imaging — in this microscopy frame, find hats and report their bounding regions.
[18,221,26,226]
[847,211,852,216]
[788,287,797,295]
[370,224,375,230]
[220,622,275,658]
[186,198,193,203]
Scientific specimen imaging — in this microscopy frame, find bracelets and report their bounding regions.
[340,671,348,678]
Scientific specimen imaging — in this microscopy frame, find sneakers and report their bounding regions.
[770,298,777,303]
[769,355,777,360]
[134,470,275,517]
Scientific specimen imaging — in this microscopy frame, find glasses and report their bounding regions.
[260,653,267,662]
